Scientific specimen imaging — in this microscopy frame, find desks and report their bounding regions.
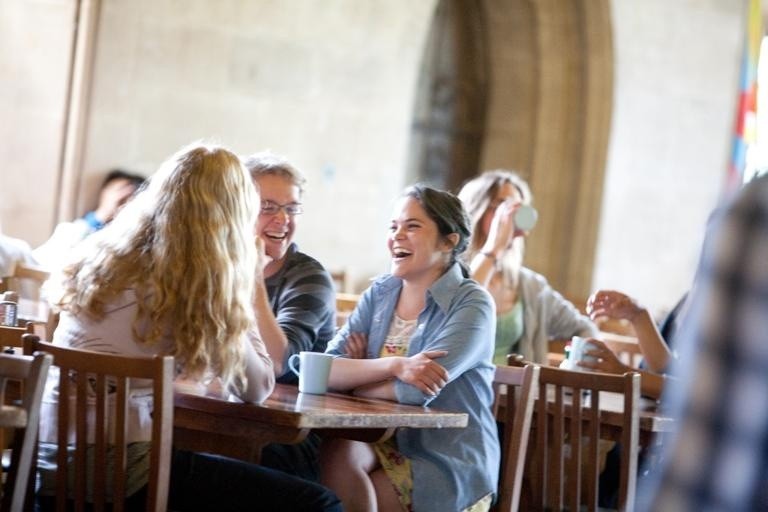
[499,377,676,447]
[173,379,469,467]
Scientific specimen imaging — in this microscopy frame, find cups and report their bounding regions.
[567,334,600,374]
[287,351,333,396]
[505,197,539,234]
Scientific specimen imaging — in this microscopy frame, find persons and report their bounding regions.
[29,171,148,283]
[324,184,500,512]
[575,291,683,402]
[37,144,342,512]
[456,172,601,391]
[242,157,335,378]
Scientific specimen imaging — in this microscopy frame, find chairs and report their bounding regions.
[0,351,54,511]
[2,264,47,301]
[506,352,641,512]
[488,363,540,512]
[21,334,175,512]
[1,322,35,354]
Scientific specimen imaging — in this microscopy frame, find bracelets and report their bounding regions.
[482,248,496,267]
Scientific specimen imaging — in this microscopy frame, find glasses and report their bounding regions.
[261,200,302,215]
